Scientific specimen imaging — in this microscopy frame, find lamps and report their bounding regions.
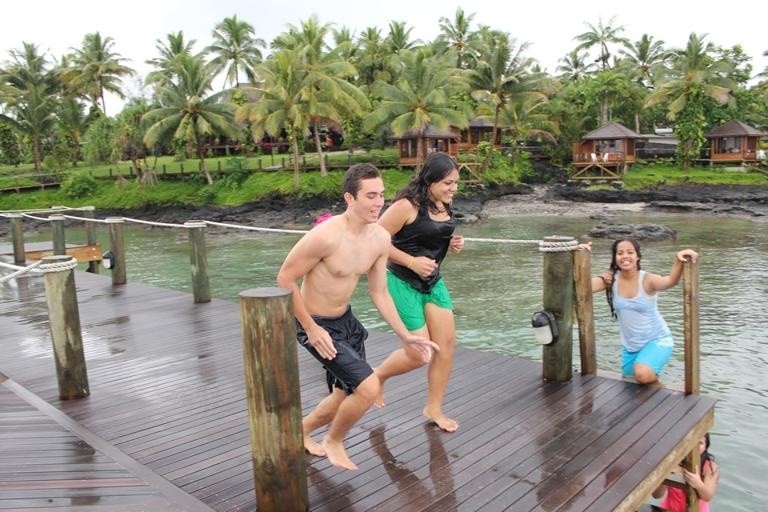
[530,309,562,347]
[102,251,115,270]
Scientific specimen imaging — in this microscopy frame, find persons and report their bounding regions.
[277,164,439,471]
[372,152,464,433]
[575,239,698,386]
[652,431,720,512]
